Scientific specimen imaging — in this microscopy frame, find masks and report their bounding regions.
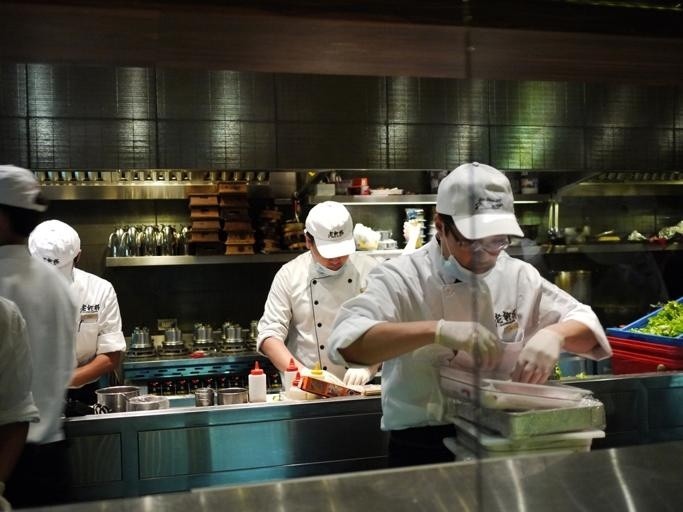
[440,253,496,285]
[314,262,347,277]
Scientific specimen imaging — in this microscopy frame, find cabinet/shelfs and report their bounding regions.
[37,180,683,270]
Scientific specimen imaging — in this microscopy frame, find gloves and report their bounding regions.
[342,363,381,385]
[511,329,564,384]
[436,318,500,370]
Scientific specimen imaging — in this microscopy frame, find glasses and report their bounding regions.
[457,236,509,254]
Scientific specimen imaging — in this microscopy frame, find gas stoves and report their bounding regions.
[119,342,282,395]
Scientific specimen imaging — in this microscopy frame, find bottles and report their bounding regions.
[195,388,212,407]
[106,224,195,257]
[283,358,306,400]
[247,360,266,402]
[304,361,324,399]
[280,198,309,251]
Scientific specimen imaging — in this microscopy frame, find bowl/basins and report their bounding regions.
[348,186,369,195]
[518,224,538,239]
[595,304,638,320]
[328,181,352,194]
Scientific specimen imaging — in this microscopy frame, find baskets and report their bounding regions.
[606,297,683,373]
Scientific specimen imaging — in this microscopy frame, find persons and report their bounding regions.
[0,162,79,505]
[325,160,614,470]
[252,199,384,394]
[0,295,40,512]
[28,218,127,415]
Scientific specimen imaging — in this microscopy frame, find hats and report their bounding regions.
[305,201,356,259]
[436,161,525,240]
[28,219,80,278]
[0,163,46,212]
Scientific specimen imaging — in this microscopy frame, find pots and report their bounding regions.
[545,270,593,306]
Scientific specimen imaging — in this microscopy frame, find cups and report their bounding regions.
[216,388,247,404]
[520,176,538,194]
[124,394,170,411]
[93,385,140,413]
[351,177,368,187]
